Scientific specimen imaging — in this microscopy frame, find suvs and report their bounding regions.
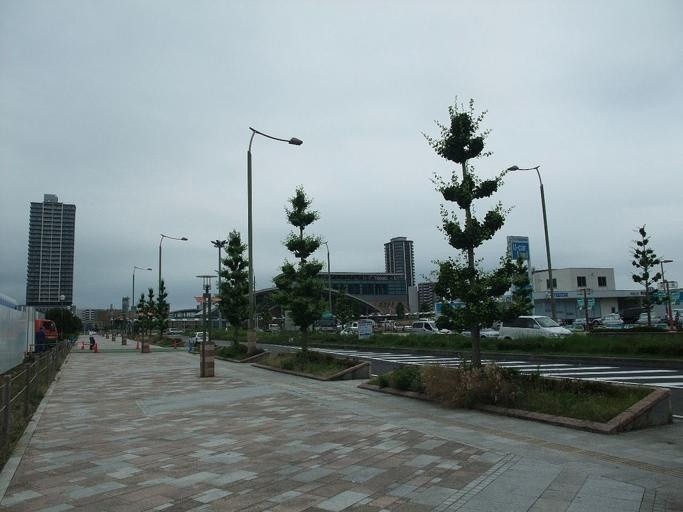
[167,327,184,335]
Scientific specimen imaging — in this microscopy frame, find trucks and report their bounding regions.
[35,319,59,351]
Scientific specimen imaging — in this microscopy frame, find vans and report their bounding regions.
[195,331,209,344]
[337,314,575,341]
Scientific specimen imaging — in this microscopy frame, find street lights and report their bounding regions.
[246,125,304,356]
[131,265,152,339]
[158,233,188,304]
[660,259,674,330]
[507,164,557,322]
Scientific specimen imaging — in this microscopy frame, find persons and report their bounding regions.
[88,335,95,350]
[34,326,45,352]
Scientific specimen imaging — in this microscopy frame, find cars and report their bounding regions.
[572,308,683,329]
